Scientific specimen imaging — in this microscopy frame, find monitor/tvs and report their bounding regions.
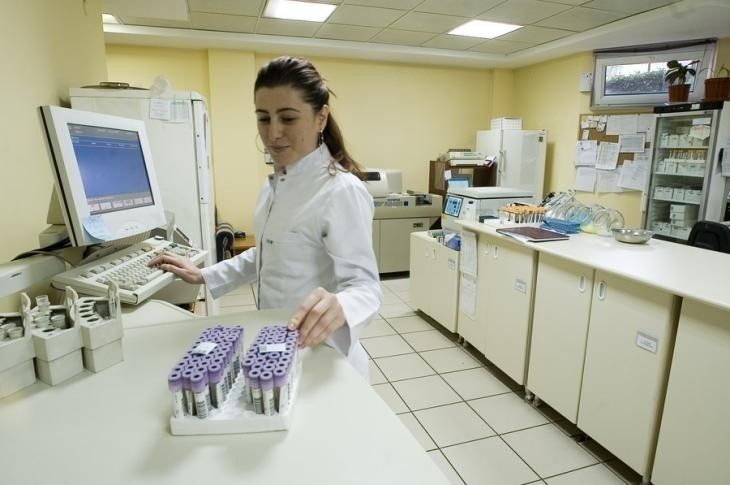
[447,178,470,188]
[37,105,166,248]
[442,195,464,218]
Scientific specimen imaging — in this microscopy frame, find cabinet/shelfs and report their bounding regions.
[638,100,719,247]
[370,190,443,274]
[454,226,538,385]
[474,129,547,203]
[526,249,684,485]
[705,112,730,228]
[408,229,461,335]
[650,298,729,485]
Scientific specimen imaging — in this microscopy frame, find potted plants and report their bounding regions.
[697,63,730,102]
[662,58,700,101]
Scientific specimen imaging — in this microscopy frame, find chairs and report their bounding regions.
[685,221,730,254]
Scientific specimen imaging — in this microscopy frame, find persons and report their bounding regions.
[147,54,383,385]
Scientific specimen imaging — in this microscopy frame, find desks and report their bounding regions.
[230,234,258,257]
[2,306,455,482]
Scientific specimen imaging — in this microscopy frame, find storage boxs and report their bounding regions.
[487,116,523,131]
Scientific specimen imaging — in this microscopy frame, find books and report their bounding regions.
[497,225,573,244]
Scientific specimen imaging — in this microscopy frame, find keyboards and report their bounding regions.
[51,236,208,306]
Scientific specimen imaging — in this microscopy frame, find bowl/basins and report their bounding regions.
[611,228,653,244]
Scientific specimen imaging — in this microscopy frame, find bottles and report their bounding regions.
[32,294,67,329]
[495,204,546,223]
[166,323,300,420]
[0,317,22,344]
[564,204,625,234]
